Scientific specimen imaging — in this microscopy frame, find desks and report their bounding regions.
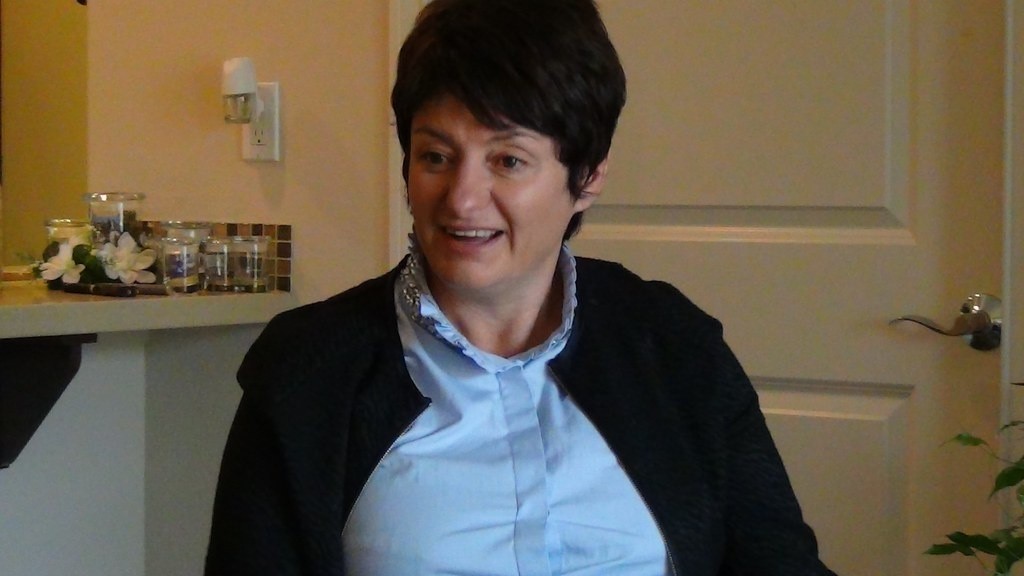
[0,279,297,468]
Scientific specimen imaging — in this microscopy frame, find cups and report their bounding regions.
[85,190,146,245]
[159,221,272,292]
[41,217,87,246]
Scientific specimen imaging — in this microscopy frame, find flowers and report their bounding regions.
[18,233,159,286]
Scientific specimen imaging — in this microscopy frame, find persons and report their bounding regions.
[202,0,833,576]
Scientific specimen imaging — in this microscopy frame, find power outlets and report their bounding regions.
[241,82,281,164]
